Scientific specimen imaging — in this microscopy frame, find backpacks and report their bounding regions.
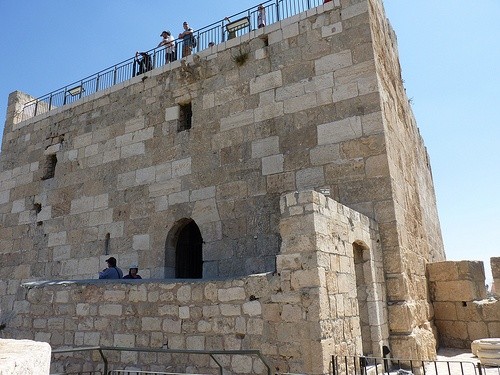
[188,28,197,48]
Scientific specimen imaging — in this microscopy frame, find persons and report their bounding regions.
[123,263,142,279]
[257,6,265,28]
[158,31,175,64]
[98,257,124,279]
[179,22,194,58]
[135,52,153,76]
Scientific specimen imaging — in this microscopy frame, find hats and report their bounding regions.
[160,31,168,36]
[183,21,188,25]
[105,257,117,265]
[129,262,138,268]
